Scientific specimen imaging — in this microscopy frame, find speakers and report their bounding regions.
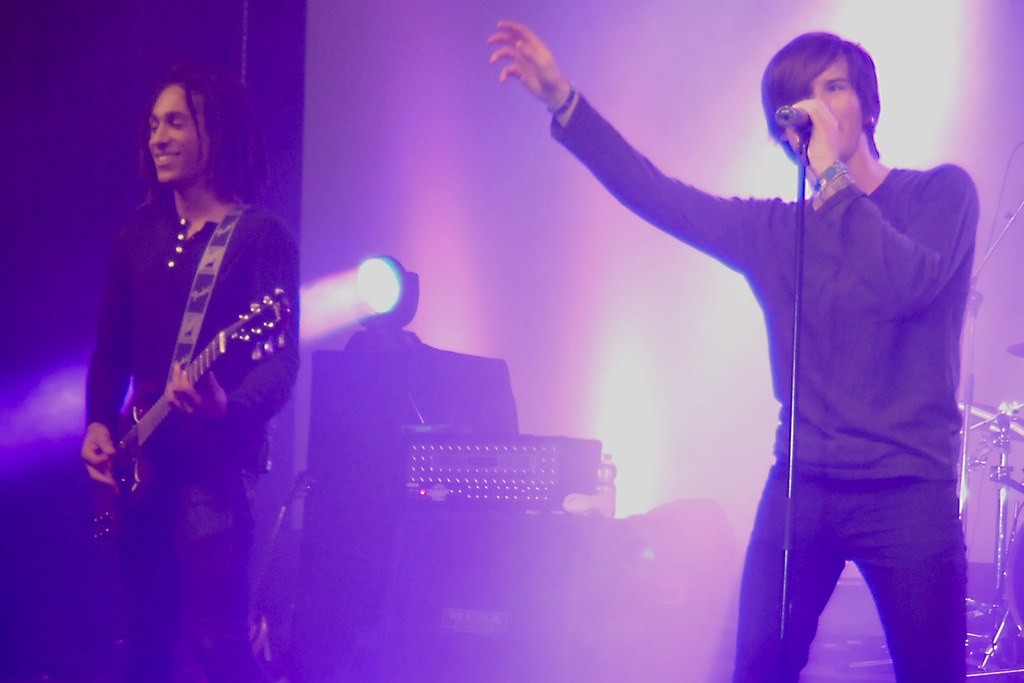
[379,505,616,683]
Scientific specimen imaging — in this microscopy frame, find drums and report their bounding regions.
[1004,521,1024,640]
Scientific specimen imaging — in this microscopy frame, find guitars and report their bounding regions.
[88,287,292,551]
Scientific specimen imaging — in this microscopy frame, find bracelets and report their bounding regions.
[815,161,854,203]
[547,84,575,117]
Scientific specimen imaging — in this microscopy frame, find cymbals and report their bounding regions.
[1005,342,1024,358]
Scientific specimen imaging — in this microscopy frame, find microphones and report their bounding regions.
[775,105,812,131]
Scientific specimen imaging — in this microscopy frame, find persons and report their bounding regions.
[487,20,981,683]
[81,67,300,683]
